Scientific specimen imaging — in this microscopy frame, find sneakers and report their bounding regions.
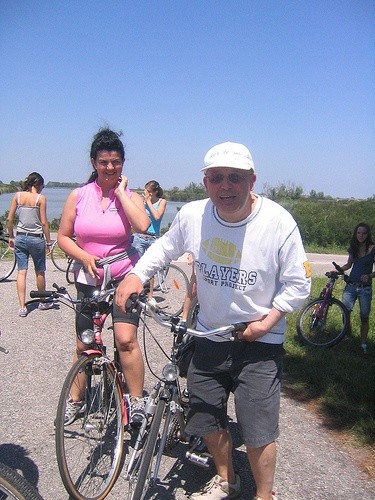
[128,396,147,425]
[53,394,88,428]
[189,472,241,500]
[18,308,28,317]
[38,302,54,310]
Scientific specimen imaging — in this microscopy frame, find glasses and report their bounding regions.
[206,175,251,183]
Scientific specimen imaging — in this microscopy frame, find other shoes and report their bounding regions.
[342,336,355,342]
[361,344,367,354]
[148,298,157,307]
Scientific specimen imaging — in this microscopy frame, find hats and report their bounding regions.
[200,142,254,176]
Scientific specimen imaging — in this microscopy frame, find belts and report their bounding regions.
[349,280,370,288]
[17,232,40,237]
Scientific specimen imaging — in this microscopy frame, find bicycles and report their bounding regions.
[294,259,357,350]
[124,285,272,500]
[0,462,44,500]
[0,222,18,283]
[21,282,167,499]
[51,233,78,284]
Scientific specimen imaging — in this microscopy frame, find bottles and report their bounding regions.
[181,389,190,415]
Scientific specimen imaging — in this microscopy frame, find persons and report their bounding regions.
[132,180,167,306]
[7,172,53,317]
[53,129,152,427]
[324,223,375,354]
[115,142,311,500]
